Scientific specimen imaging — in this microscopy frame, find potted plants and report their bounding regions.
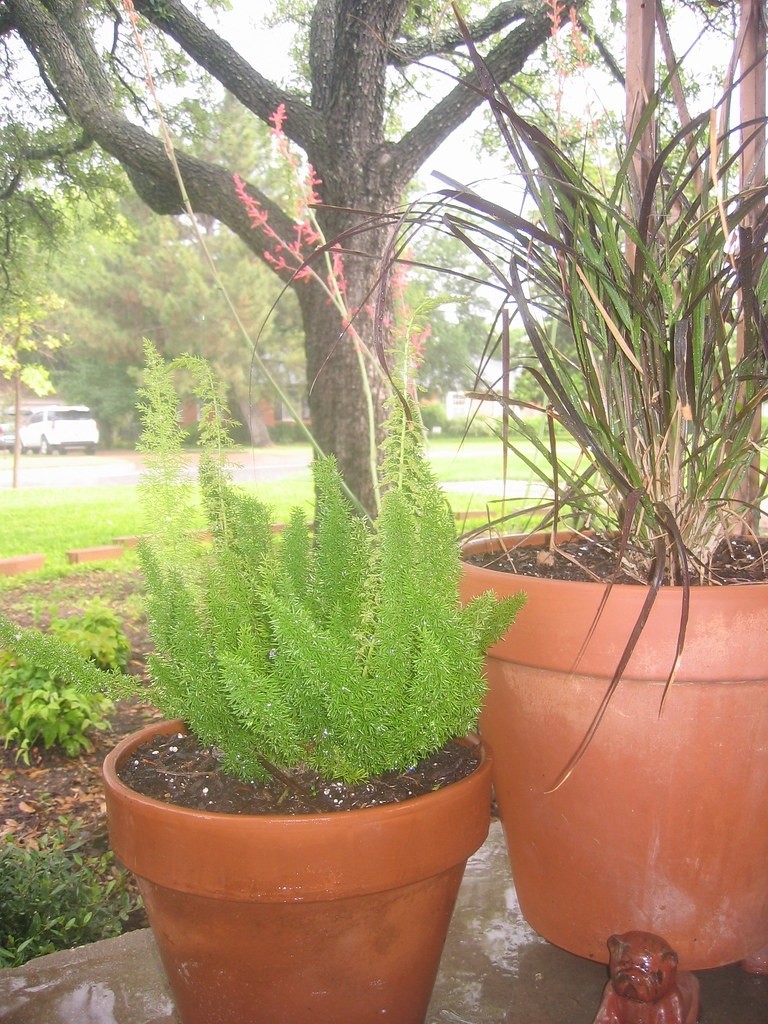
[258,0,768,977]
[85,0,527,1024]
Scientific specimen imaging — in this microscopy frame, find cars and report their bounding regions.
[21,405,101,456]
[1,404,46,452]
